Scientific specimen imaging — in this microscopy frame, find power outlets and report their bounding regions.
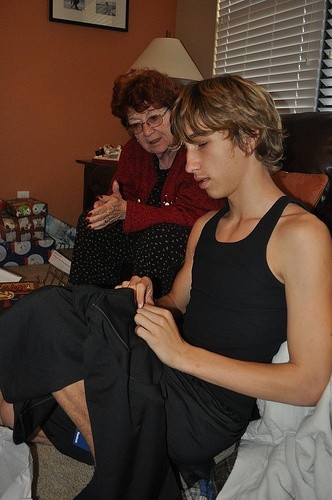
[17,190,30,199]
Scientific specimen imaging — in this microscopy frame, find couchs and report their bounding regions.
[256,111,332,239]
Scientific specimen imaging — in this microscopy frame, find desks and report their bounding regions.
[75,157,118,213]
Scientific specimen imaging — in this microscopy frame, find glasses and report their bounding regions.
[126,106,169,136]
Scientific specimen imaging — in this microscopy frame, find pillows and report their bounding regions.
[270,169,329,214]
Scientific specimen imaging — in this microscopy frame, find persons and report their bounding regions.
[0,74,331,500]
[68,70,227,298]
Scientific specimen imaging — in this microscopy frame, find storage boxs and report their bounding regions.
[0,198,56,268]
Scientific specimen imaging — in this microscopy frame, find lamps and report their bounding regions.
[124,30,205,86]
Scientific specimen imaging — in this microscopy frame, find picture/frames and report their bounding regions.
[49,0,129,33]
